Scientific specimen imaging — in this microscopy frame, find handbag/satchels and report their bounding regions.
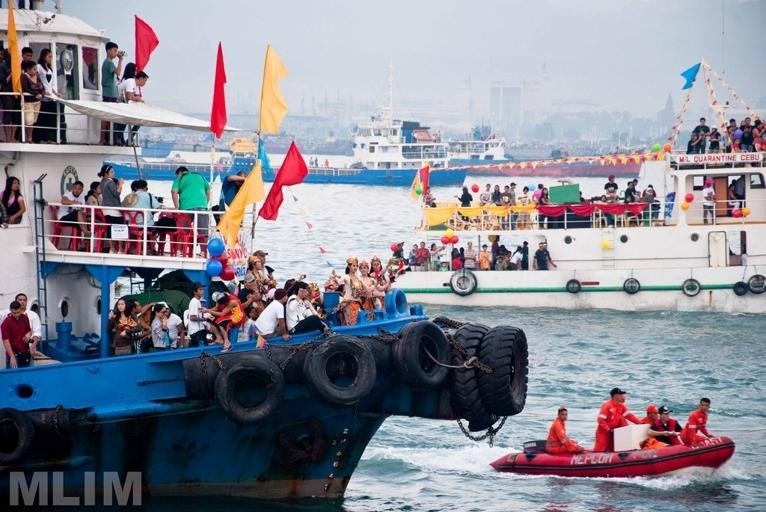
[12,349,32,368]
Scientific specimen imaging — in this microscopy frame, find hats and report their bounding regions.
[395,239,405,246]
[610,387,627,395]
[346,258,359,264]
[191,281,208,291]
[510,182,517,187]
[244,272,256,284]
[247,249,269,265]
[712,125,718,129]
[705,178,715,185]
[658,404,673,415]
[646,404,660,414]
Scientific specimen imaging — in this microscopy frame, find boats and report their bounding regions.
[485,432,737,476]
[0,2,529,509]
[387,151,766,313]
[445,117,669,177]
[102,64,467,188]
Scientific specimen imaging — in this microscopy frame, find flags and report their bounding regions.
[135,17,159,75]
[4,1,23,97]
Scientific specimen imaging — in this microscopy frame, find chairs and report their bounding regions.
[48,200,208,258]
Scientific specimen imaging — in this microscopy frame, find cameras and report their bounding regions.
[121,50,128,57]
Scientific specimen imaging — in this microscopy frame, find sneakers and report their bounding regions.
[323,328,337,339]
[109,246,125,255]
[220,341,233,355]
[206,340,225,346]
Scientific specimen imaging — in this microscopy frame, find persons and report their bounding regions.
[545,408,583,454]
[396,116,766,273]
[2,40,396,366]
[594,387,713,451]
